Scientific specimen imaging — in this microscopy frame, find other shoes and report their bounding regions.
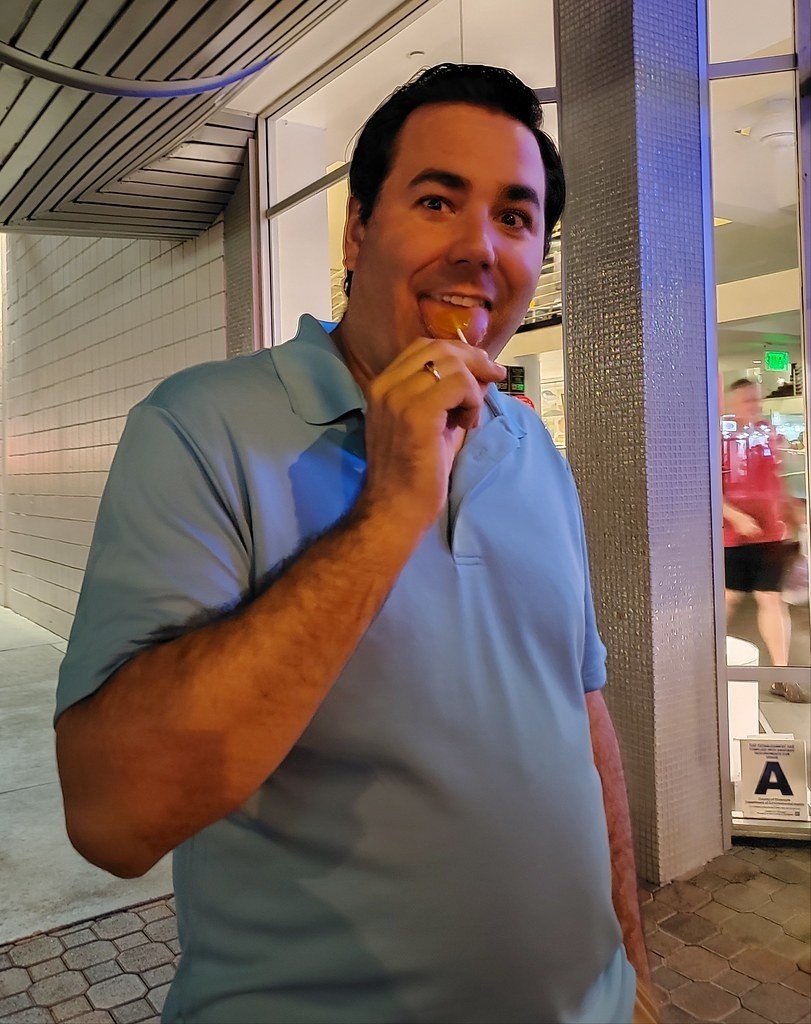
[769,681,807,703]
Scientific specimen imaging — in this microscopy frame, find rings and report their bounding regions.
[424,361,441,381]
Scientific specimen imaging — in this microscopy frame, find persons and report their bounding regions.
[722,380,810,704]
[55,66,654,1023]
